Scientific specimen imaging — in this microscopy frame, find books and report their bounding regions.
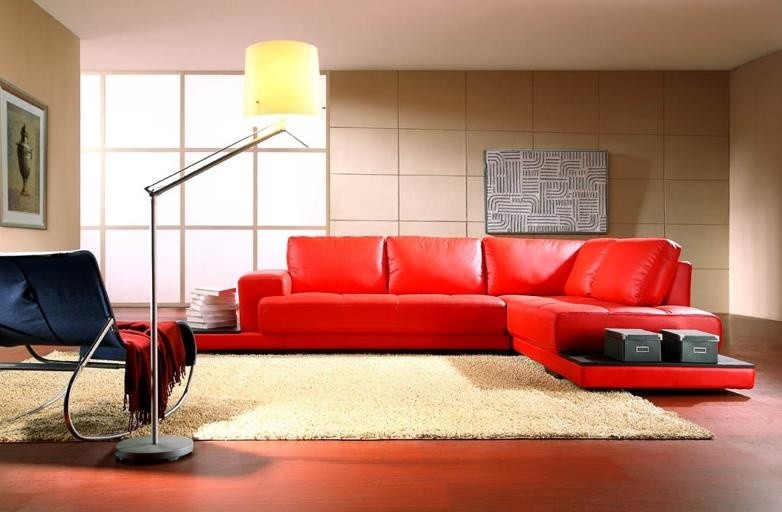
[185,285,237,329]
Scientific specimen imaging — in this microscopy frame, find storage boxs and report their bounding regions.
[659,328,721,364]
[604,327,663,364]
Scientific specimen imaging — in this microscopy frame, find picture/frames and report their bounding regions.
[0,76,49,231]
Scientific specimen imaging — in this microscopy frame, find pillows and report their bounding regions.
[561,237,681,308]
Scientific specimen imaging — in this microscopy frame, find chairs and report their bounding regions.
[0,249,197,444]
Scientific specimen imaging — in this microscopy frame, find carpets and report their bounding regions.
[0,348,718,441]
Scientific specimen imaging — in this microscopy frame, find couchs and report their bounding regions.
[192,234,512,351]
[481,235,757,391]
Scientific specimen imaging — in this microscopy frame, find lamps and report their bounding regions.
[116,39,323,465]
